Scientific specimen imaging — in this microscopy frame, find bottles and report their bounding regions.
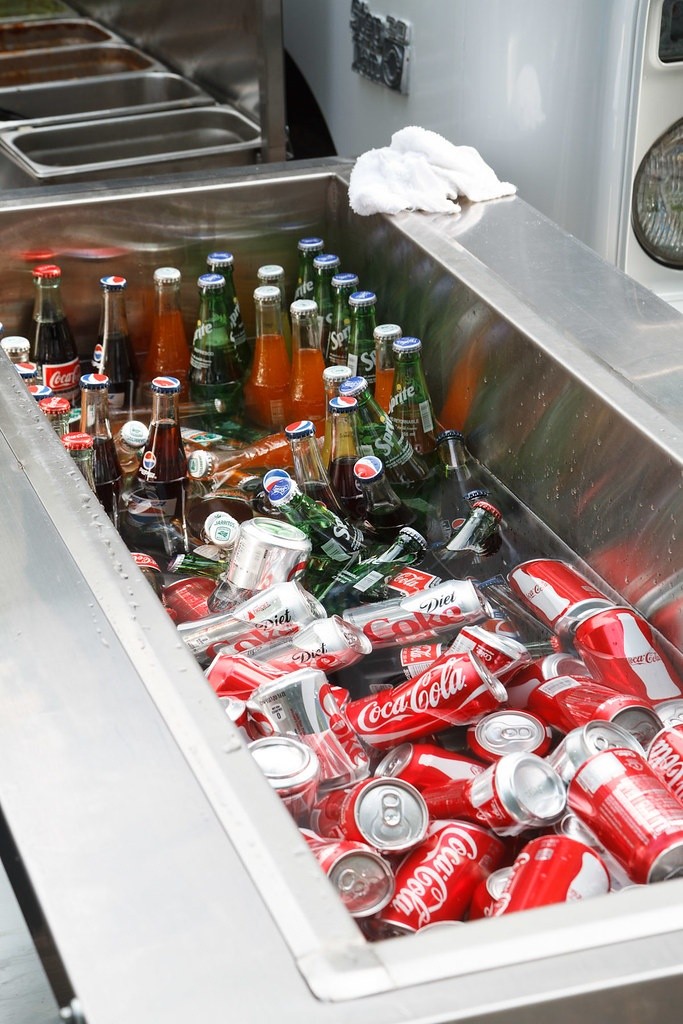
[0,235,522,675]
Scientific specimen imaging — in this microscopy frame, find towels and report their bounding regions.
[347,123,518,216]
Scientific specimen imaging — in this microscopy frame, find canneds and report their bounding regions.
[158,518,683,943]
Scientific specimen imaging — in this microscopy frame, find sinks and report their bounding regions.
[0,173,683,944]
[0,76,207,120]
[0,47,158,87]
[10,109,262,168]
[0,22,114,52]
